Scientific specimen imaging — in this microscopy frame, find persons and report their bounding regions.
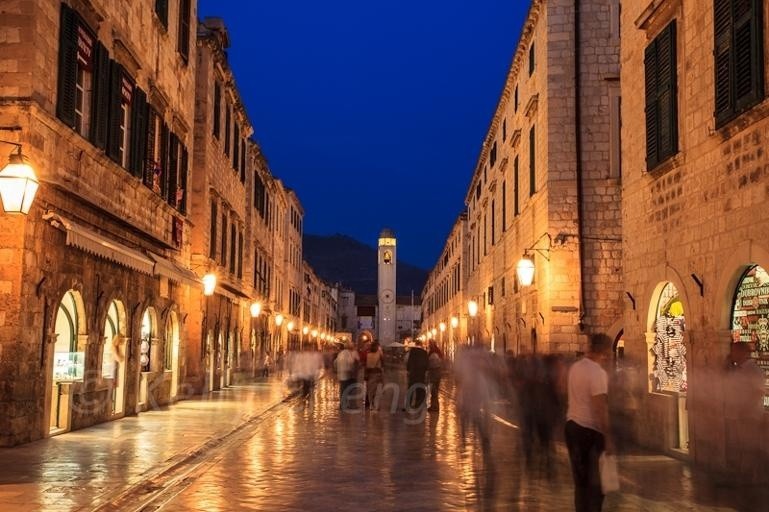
[263,332,643,509]
[712,340,766,490]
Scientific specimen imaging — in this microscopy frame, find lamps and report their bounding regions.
[0,138,40,216]
[516,246,549,286]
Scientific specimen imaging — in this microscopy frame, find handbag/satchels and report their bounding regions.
[599,450,620,492]
